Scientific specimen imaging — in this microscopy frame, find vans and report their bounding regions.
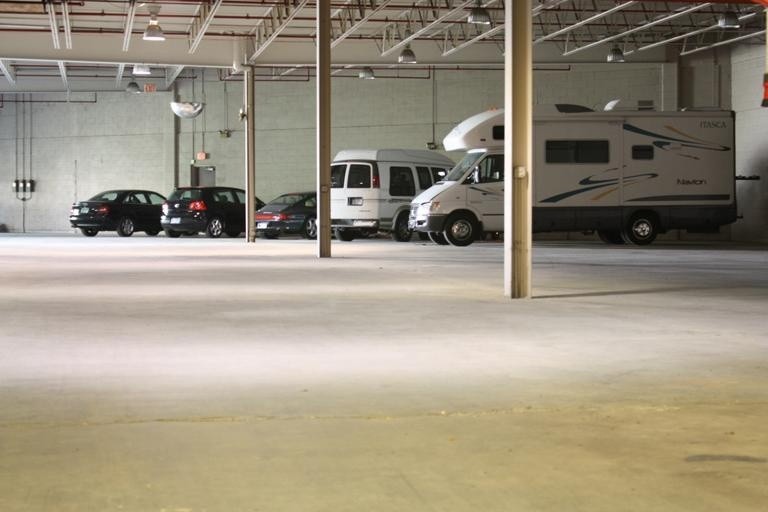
[405,94,740,245]
[330,147,457,241]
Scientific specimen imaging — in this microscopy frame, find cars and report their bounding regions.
[70,189,170,236]
[161,183,266,239]
[254,186,317,240]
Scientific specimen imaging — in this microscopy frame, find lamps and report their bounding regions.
[715,2,742,28]
[397,29,416,65]
[468,0,492,26]
[359,67,374,79]
[606,41,626,65]
[141,6,167,44]
